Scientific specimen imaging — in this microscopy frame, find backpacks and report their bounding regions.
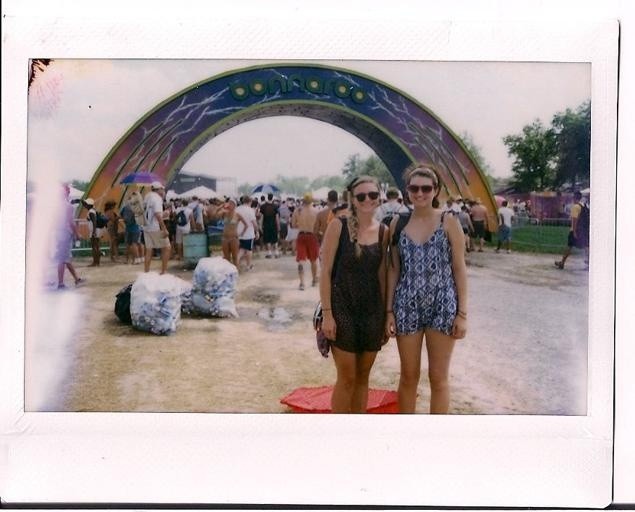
[127,191,154,226]
[174,207,190,225]
[575,202,589,241]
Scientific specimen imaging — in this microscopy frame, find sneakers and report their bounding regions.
[244,264,257,274]
[494,248,500,254]
[133,257,146,265]
[507,249,512,254]
[299,276,319,290]
[57,284,69,290]
[555,261,564,269]
[75,277,87,288]
[265,254,282,259]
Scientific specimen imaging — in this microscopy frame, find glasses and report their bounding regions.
[352,192,381,203]
[406,186,436,193]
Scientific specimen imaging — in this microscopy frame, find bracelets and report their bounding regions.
[458,313,467,320]
[321,308,331,311]
[459,310,467,316]
[388,309,393,314]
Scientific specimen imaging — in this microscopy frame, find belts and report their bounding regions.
[298,230,311,234]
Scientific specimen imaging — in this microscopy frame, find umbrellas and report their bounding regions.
[119,171,163,186]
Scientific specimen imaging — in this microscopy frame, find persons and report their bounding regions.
[84,180,348,291]
[319,175,390,413]
[554,191,589,269]
[513,198,531,216]
[374,188,413,226]
[385,163,468,415]
[57,182,88,290]
[441,195,515,255]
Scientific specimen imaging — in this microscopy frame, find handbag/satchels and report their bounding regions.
[484,231,492,243]
[115,285,132,322]
[313,299,330,358]
[116,218,126,233]
[95,212,110,228]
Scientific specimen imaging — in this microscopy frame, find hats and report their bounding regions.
[81,197,96,209]
[151,182,165,190]
